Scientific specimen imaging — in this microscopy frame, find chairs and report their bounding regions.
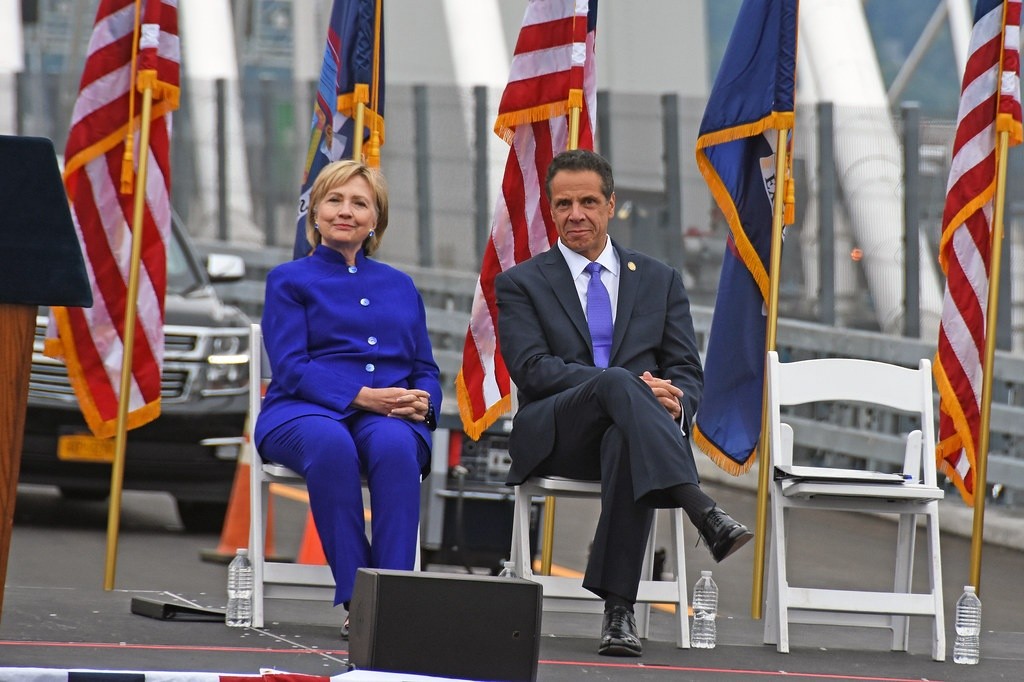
[761,350,946,662]
[244,324,423,628]
[508,375,693,651]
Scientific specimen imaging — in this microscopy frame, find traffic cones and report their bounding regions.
[198,383,297,567]
[296,500,330,566]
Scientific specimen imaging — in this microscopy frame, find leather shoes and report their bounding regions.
[695,502,754,563]
[597,606,643,657]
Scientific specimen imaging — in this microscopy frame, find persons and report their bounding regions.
[493,150,757,656]
[253,161,443,634]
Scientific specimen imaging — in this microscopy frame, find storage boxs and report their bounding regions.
[347,567,544,682]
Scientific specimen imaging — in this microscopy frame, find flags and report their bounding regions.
[689,3,796,476]
[453,1,599,440]
[42,3,181,439]
[931,2,1022,507]
[291,1,384,259]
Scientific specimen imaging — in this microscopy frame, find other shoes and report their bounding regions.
[340,614,349,640]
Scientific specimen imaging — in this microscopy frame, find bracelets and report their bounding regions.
[425,399,433,423]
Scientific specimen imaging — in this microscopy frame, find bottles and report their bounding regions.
[498,561,519,579]
[225,548,253,628]
[690,571,719,650]
[953,585,981,665]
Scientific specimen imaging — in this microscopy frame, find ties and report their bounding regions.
[584,262,614,368]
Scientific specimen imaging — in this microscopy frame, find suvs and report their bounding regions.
[12,202,273,530]
